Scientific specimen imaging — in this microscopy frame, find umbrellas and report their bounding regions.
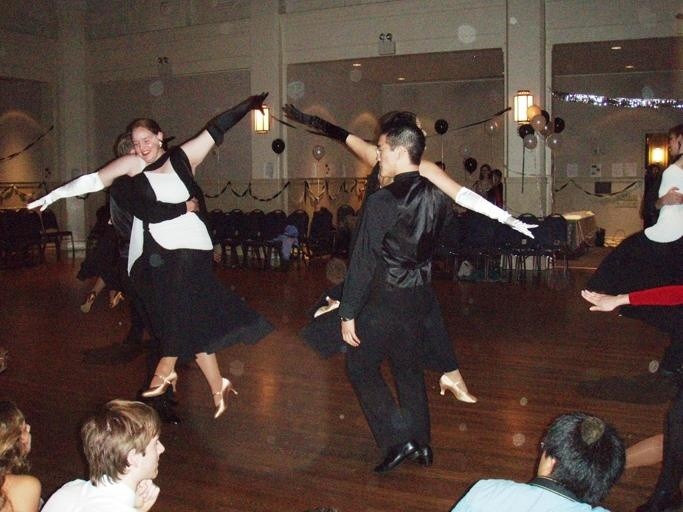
[417,446,433,467]
[374,438,422,472]
[634,491,681,512]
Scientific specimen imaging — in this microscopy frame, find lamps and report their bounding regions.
[431,213,571,289]
[1,208,75,271]
[192,205,361,274]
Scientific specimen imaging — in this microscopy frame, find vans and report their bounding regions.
[80,291,98,313]
[439,373,478,403]
[213,377,239,420]
[108,292,125,309]
[313,296,340,318]
[142,371,179,398]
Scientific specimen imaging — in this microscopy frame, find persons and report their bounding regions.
[584,152,682,376]
[448,409,625,511]
[337,121,454,474]
[0,397,42,512]
[649,123,682,210]
[579,283,683,312]
[106,131,201,425]
[74,200,128,314]
[280,102,539,406]
[37,397,165,512]
[625,385,682,512]
[435,157,503,229]
[25,89,270,420]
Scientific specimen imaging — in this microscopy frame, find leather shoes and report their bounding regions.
[26,171,105,212]
[281,102,351,145]
[205,91,269,147]
[454,185,539,240]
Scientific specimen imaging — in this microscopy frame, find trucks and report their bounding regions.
[271,138,284,155]
[485,117,499,135]
[517,104,564,150]
[457,144,472,159]
[434,119,447,135]
[312,145,325,161]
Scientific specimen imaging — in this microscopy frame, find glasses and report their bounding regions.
[255,104,270,133]
[513,90,534,125]
